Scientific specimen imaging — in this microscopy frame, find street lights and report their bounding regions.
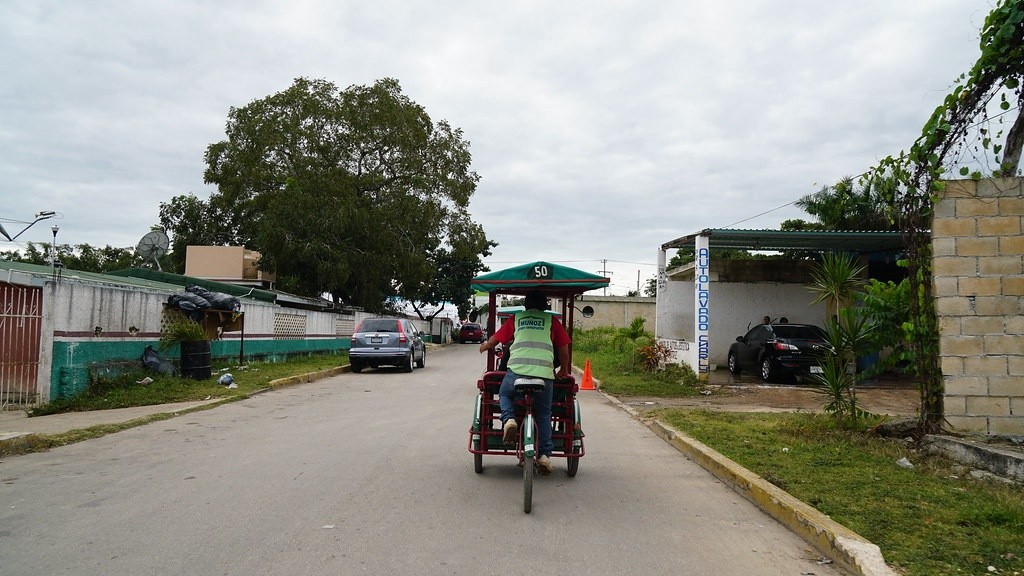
[50,226,60,280]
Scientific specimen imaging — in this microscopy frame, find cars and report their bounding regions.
[728,323,847,384]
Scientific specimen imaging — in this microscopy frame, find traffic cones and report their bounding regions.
[579,360,597,390]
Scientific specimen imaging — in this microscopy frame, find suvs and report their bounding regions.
[348,316,426,372]
[459,323,484,344]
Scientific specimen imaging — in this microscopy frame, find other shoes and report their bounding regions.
[538,455,553,473]
[502,418,517,444]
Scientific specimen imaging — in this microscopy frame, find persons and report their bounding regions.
[764,316,789,324]
[479,292,571,476]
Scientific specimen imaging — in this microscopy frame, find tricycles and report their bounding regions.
[467,262,611,514]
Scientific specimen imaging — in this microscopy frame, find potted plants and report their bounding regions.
[157,314,211,380]
